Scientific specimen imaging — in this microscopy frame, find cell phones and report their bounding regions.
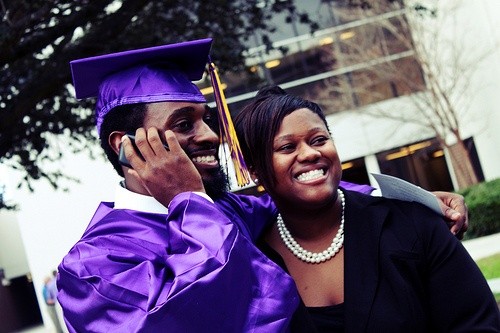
[119,134,170,169]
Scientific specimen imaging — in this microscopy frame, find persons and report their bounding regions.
[232,82,500,333]
[43,271,69,333]
[54,38,470,333]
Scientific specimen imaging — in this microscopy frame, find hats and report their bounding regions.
[70,38,251,188]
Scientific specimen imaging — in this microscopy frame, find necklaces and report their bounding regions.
[277,189,345,264]
